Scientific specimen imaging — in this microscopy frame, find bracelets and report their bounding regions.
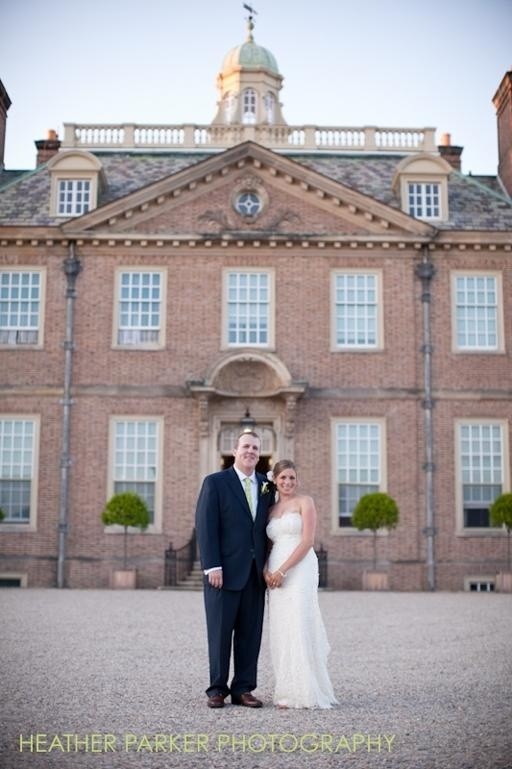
[278,569,287,578]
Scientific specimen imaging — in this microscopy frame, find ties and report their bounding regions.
[243,478,253,513]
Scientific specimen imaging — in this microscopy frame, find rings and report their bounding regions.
[272,581,276,585]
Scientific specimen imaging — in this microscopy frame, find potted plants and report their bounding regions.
[351,493,398,592]
[101,492,150,589]
[489,493,511,594]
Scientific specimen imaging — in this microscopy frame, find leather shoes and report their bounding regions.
[206,694,224,708]
[230,692,263,708]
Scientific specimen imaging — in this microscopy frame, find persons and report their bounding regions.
[261,458,318,710]
[194,431,278,710]
